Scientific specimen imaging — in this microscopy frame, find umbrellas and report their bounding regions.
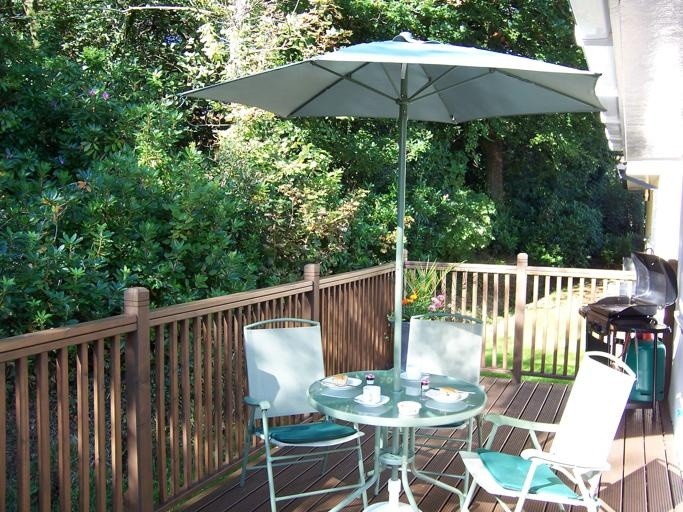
[177,30,609,512]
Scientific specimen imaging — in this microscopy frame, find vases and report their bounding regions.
[393,321,409,372]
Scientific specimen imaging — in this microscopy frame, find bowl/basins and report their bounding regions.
[397,401,422,416]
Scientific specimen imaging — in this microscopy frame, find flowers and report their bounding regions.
[387,255,468,319]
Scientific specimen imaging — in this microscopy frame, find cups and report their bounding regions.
[364,386,381,403]
[404,386,420,396]
[406,366,421,380]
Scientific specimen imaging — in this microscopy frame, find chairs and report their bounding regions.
[458,350,637,512]
[374,313,486,497]
[239,318,368,512]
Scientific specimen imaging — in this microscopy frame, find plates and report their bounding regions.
[426,388,469,404]
[322,389,360,399]
[427,401,469,413]
[355,397,390,407]
[321,375,362,391]
[400,372,422,381]
[357,407,392,415]
